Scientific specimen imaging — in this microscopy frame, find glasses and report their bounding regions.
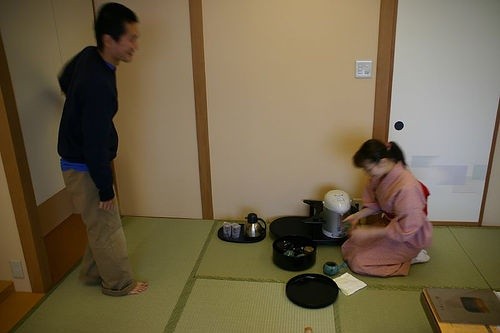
[363,162,376,173]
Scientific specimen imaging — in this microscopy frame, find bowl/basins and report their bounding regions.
[273,235,317,271]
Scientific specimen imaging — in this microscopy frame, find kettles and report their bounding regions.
[244,213,266,239]
[322,189,352,238]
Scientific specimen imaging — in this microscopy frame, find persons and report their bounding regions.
[340,138,432,278]
[54,1,151,296]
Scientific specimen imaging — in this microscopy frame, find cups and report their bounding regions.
[223,222,231,237]
[232,223,241,239]
[324,261,338,276]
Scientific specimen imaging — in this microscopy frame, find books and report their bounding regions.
[424,287,500,325]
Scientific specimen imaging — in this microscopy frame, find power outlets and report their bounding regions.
[356,60,372,79]
[11,259,23,278]
